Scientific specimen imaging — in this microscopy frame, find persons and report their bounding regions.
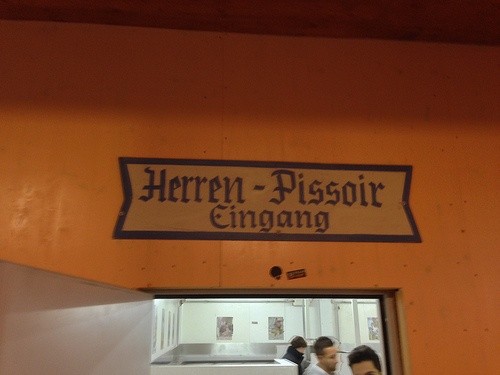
[347,345,382,375]
[307,336,339,375]
[282,336,307,375]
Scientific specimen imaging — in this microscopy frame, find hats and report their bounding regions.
[290,336,307,349]
